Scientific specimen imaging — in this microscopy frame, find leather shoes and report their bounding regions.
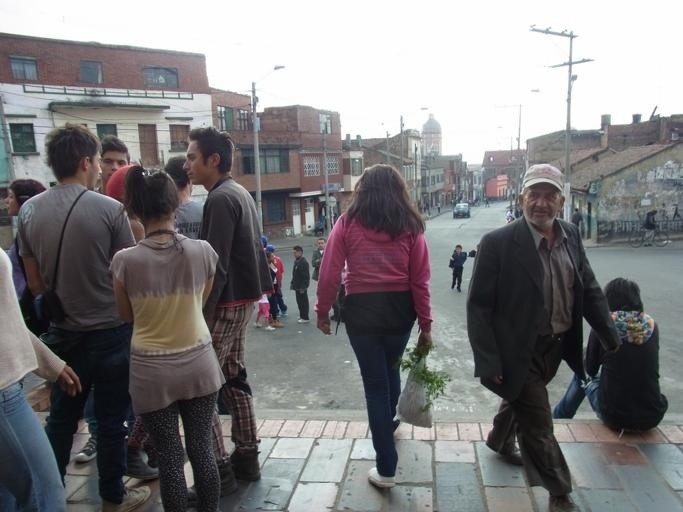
[487,432,522,463]
[548,494,582,512]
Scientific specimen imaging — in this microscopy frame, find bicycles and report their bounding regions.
[627,223,669,248]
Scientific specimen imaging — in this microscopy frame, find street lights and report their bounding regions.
[515,86,544,197]
[397,105,432,185]
[562,74,579,220]
[250,64,288,234]
[497,125,514,213]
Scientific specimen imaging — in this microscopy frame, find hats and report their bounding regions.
[523,163,565,192]
[262,236,274,254]
[107,166,134,200]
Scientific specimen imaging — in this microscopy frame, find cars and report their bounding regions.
[451,203,472,218]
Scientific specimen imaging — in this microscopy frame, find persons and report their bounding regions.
[1,122,277,511]
[254,234,342,332]
[642,209,657,246]
[507,209,514,225]
[553,277,669,434]
[448,245,467,292]
[466,164,622,512]
[315,165,433,490]
[573,208,583,224]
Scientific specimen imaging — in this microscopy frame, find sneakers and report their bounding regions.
[75,437,97,462]
[230,447,260,480]
[103,446,160,511]
[188,465,238,505]
[368,467,396,488]
